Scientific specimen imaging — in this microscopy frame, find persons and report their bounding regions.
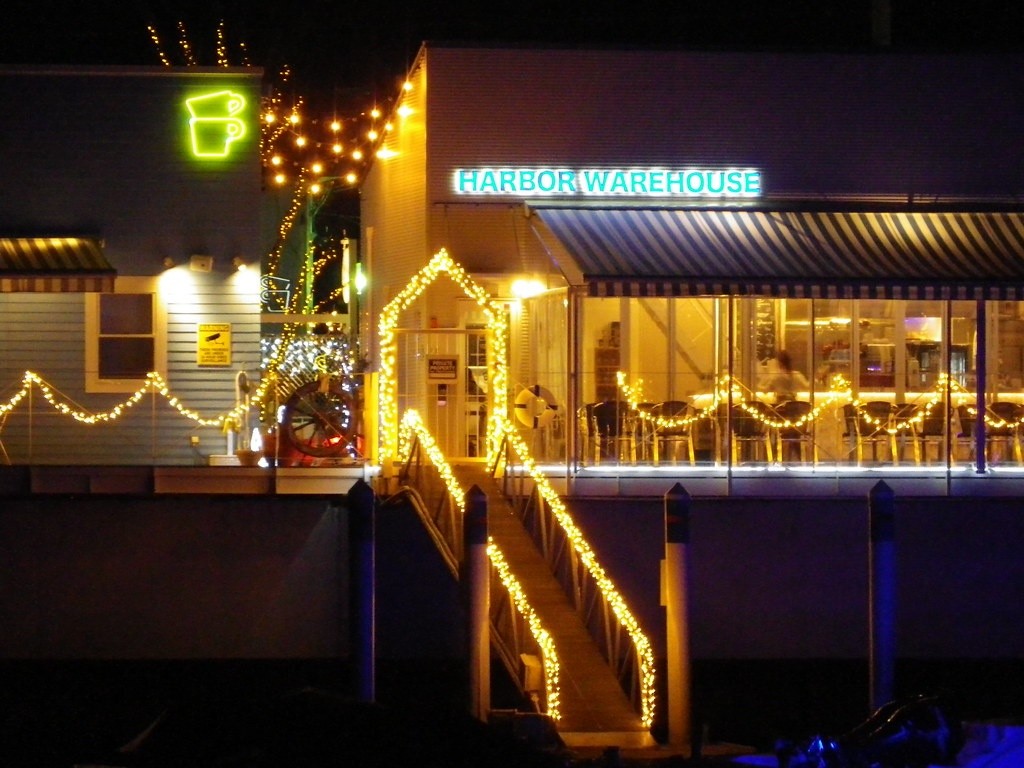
[758,349,828,462]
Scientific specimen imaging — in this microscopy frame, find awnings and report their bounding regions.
[0,236,116,295]
[533,209,1024,300]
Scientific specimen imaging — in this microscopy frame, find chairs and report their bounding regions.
[622,399,1023,462]
